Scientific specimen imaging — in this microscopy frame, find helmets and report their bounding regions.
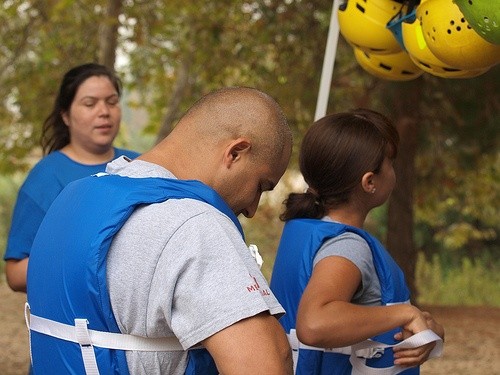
[337,0,500,81]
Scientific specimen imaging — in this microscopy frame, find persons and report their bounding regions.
[25,85,296,375]
[2,63,143,292]
[269,108,445,374]
